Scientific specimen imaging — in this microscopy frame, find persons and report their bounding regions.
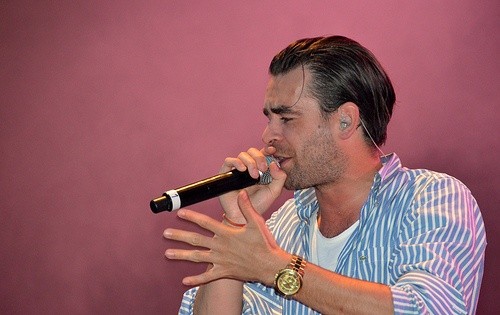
[164,34,487,315]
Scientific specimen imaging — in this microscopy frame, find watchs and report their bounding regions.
[273,254,306,300]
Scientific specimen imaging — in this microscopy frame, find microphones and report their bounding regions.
[150,155,280,213]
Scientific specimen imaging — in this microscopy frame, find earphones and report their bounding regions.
[340,117,351,128]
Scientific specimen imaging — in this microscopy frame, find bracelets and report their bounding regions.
[224,215,247,228]
